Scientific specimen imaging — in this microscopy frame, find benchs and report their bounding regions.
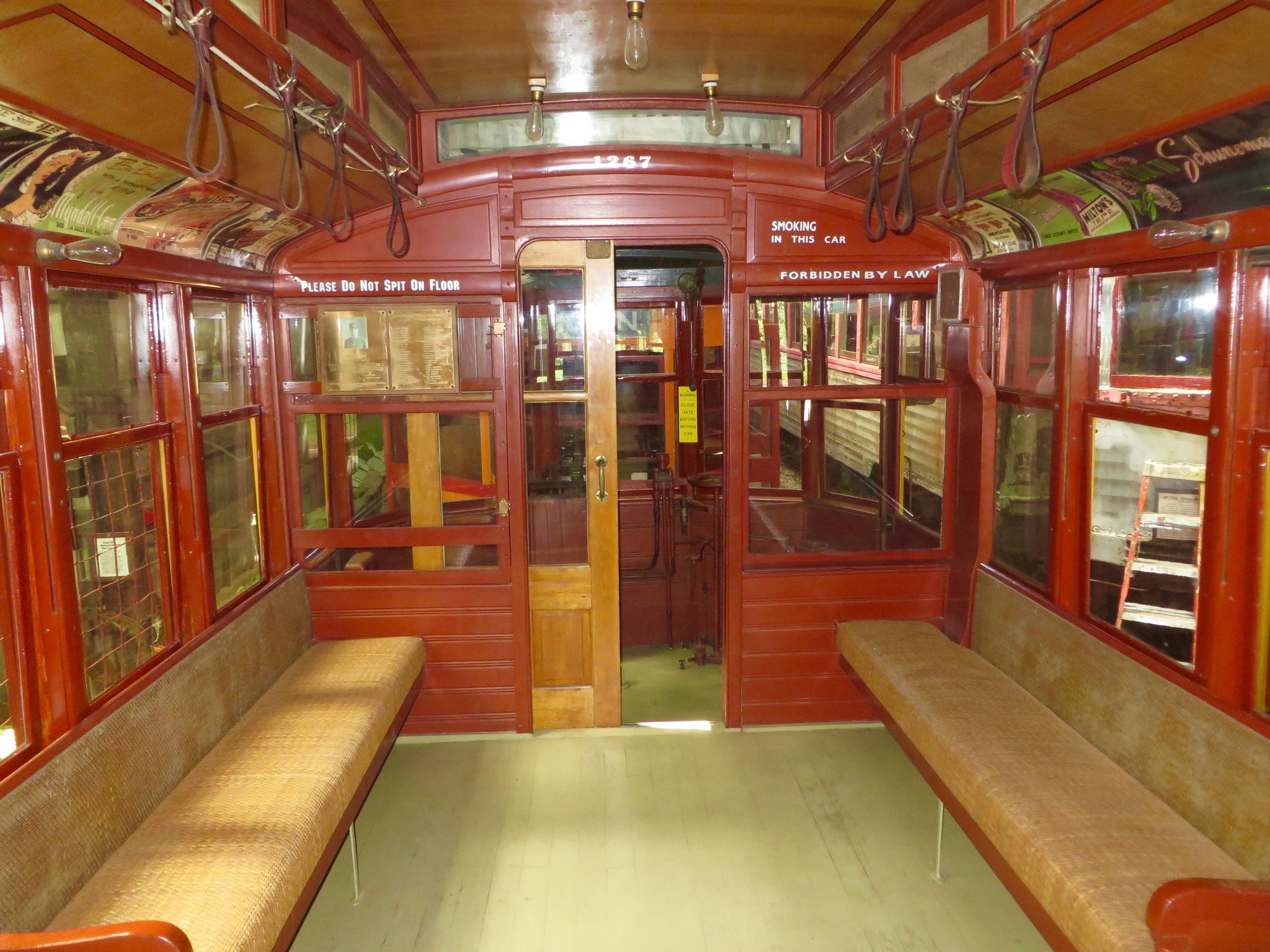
[835,563,1270,952]
[0,565,426,952]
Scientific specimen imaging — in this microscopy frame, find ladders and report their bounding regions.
[1115,459,1206,670]
